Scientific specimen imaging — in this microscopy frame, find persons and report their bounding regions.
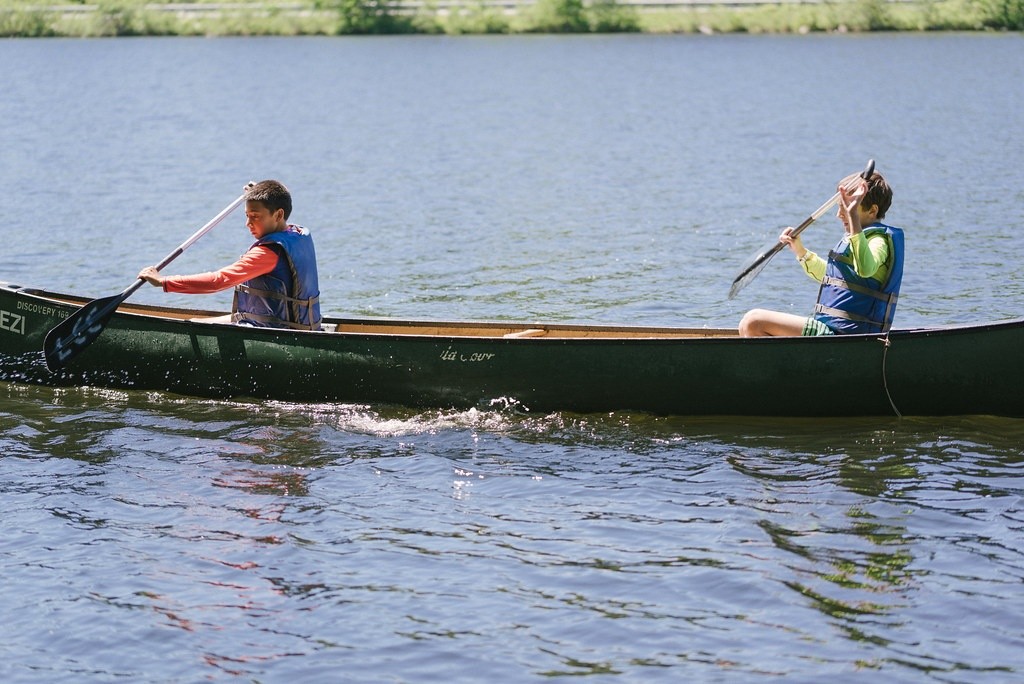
[739,169,904,339]
[138,180,321,330]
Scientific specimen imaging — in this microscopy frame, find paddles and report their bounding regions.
[728,158,875,302]
[42,181,254,375]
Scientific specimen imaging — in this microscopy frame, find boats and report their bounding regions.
[0,282,1024,417]
